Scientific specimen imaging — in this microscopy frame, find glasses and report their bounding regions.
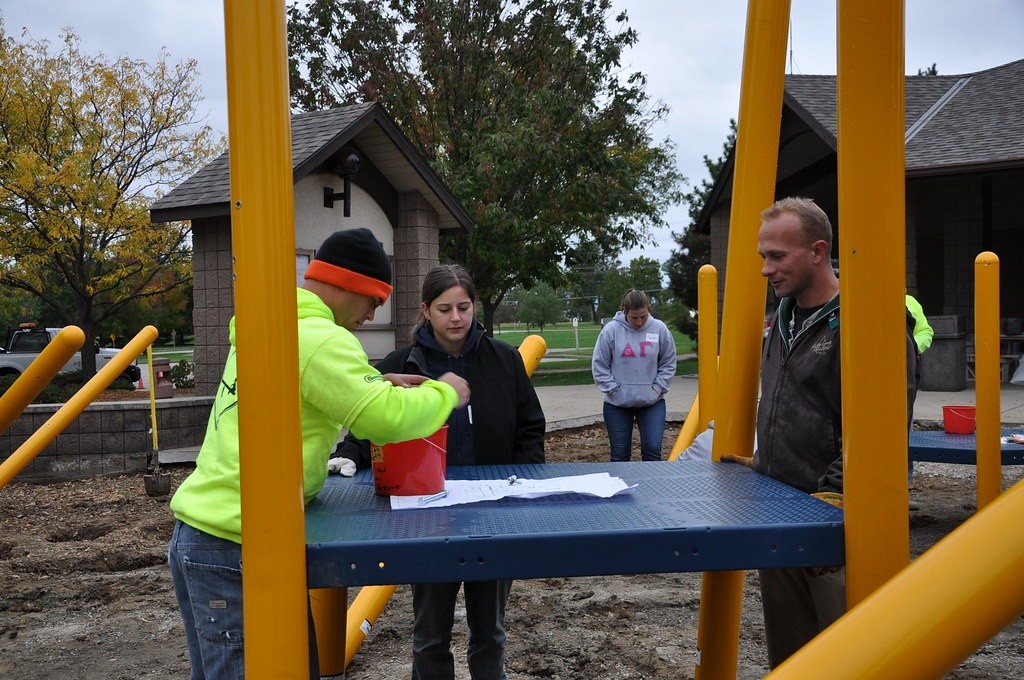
[369,297,381,309]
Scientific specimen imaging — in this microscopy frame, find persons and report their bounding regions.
[591,288,677,462]
[327,263,547,679]
[905,294,934,509]
[169,227,472,680]
[719,196,918,672]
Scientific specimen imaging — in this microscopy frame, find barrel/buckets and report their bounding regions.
[942,406,975,434]
[371,423,449,496]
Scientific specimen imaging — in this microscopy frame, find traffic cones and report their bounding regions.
[138,378,147,389]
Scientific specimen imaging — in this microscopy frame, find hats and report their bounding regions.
[304,228,394,306]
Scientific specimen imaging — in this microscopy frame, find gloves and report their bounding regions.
[327,456,357,477]
[720,452,755,469]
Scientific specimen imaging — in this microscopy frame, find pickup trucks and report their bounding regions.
[0,323,141,384]
